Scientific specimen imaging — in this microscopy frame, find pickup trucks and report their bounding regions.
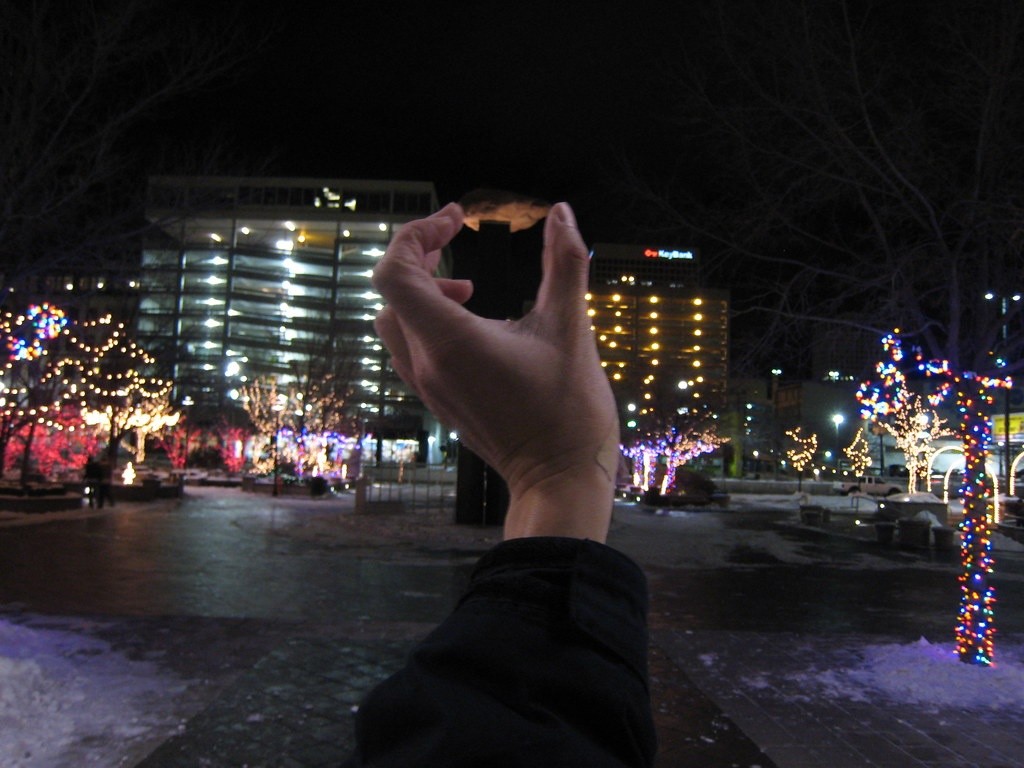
[832,474,904,498]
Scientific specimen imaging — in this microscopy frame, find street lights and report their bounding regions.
[181,394,195,470]
[833,414,844,474]
[270,401,286,496]
[983,291,1022,498]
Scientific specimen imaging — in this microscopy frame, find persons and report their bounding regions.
[349,200,657,768]
[84,455,115,509]
